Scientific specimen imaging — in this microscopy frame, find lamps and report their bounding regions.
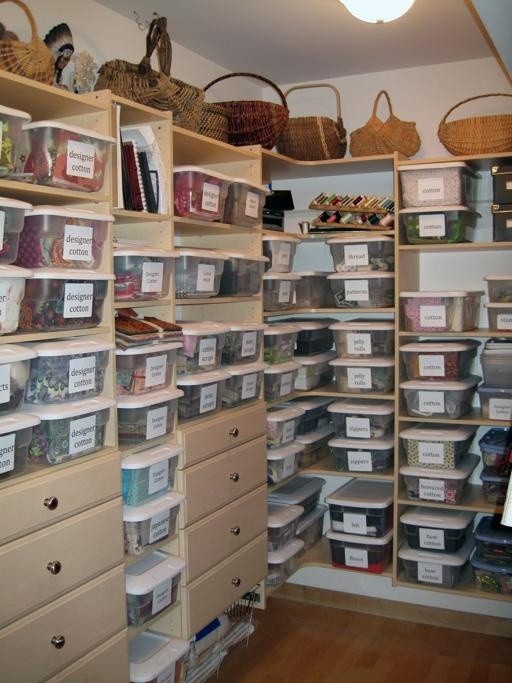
[340,0,414,24]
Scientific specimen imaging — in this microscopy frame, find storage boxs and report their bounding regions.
[295,429,335,467]
[174,249,229,299]
[267,501,304,552]
[325,478,393,538]
[473,517,511,566]
[268,540,304,580]
[176,370,231,421]
[263,236,301,272]
[329,322,395,359]
[129,630,189,683]
[328,357,395,394]
[297,271,332,308]
[470,548,511,595]
[224,179,270,227]
[327,401,395,440]
[326,274,395,309]
[0,344,38,418]
[492,204,512,242]
[399,206,473,243]
[222,363,269,407]
[328,437,394,472]
[19,268,115,331]
[399,376,482,418]
[264,273,301,309]
[483,273,512,303]
[1,266,33,334]
[1,105,31,179]
[114,249,177,301]
[14,205,115,269]
[398,162,481,207]
[399,291,485,332]
[220,251,270,297]
[399,339,480,381]
[296,505,328,549]
[480,337,512,387]
[118,389,183,445]
[267,403,305,448]
[0,415,39,479]
[399,454,480,505]
[400,508,476,553]
[478,428,511,476]
[265,362,301,400]
[264,325,301,364]
[123,492,184,555]
[295,322,336,356]
[222,324,269,364]
[480,466,512,505]
[176,321,230,375]
[326,529,393,574]
[490,163,512,204]
[121,442,183,507]
[24,342,115,404]
[117,344,182,396]
[477,384,512,420]
[1,196,32,265]
[483,303,512,331]
[173,166,233,220]
[399,425,478,469]
[295,400,336,434]
[25,398,115,465]
[268,443,303,482]
[269,477,325,520]
[294,351,336,391]
[397,539,476,587]
[326,238,394,273]
[23,121,113,193]
[126,551,185,627]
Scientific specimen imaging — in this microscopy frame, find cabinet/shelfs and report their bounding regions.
[181,401,267,641]
[397,153,512,603]
[0,71,127,484]
[0,450,130,683]
[262,149,397,590]
[170,127,267,428]
[110,92,187,682]
